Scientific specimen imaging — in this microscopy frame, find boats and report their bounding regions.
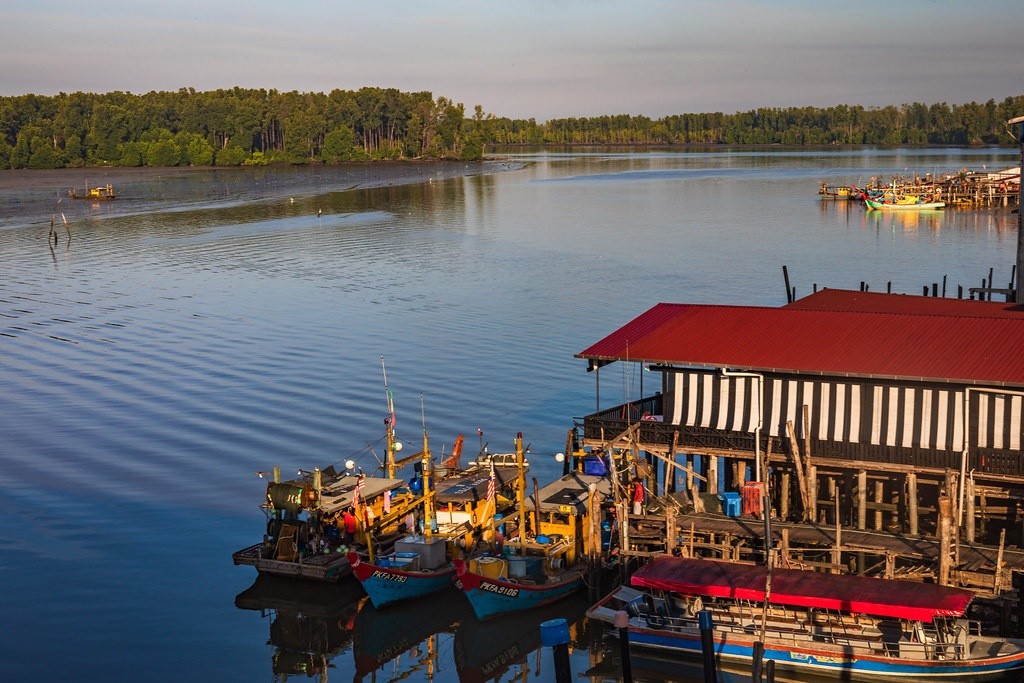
[816,162,1021,212]
[452,427,645,623]
[583,546,1024,683]
[344,427,532,609]
[67,176,116,201]
[232,355,435,584]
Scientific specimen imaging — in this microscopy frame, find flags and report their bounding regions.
[485,471,496,502]
[350,472,366,508]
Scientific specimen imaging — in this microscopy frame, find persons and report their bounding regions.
[342,508,355,546]
[631,476,643,514]
[328,522,340,546]
